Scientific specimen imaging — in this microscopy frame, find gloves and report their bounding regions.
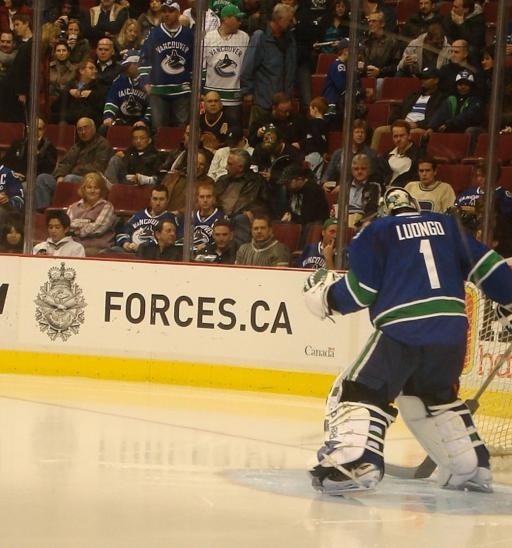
[302,267,342,321]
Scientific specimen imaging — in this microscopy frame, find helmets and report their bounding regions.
[376,187,421,217]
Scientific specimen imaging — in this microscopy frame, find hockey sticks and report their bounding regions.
[385,342,512,479]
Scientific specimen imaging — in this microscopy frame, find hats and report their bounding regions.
[454,68,476,84]
[321,218,338,231]
[222,125,244,145]
[118,49,141,66]
[159,0,181,13]
[337,37,364,49]
[414,63,444,79]
[220,4,248,18]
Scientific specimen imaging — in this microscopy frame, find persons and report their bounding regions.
[0,0,512,269]
[300,182,512,496]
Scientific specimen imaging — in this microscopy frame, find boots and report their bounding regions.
[434,405,492,482]
[310,401,385,482]
